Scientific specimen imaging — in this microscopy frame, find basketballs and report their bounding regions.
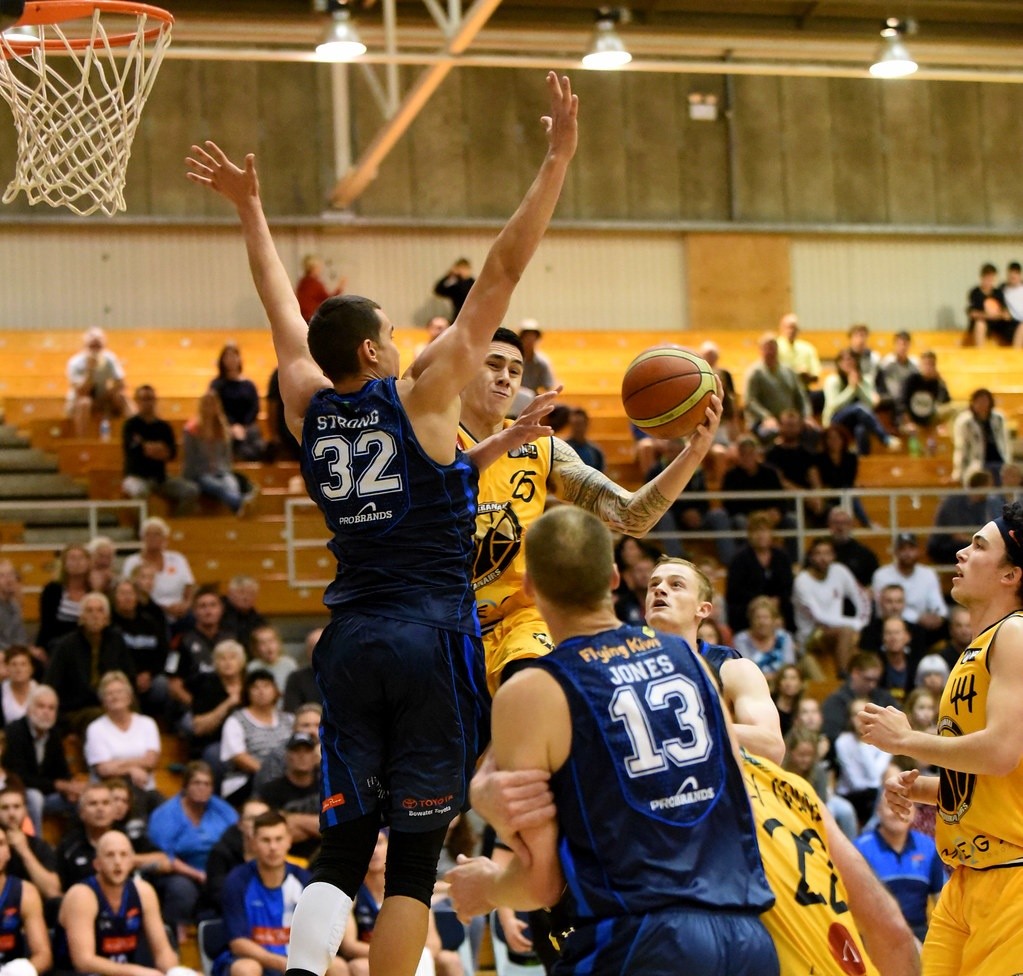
[620,343,720,441]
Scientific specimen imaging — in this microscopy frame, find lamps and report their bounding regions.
[313,0,367,60]
[687,91,717,121]
[580,3,632,68]
[1,25,41,43]
[868,16,919,77]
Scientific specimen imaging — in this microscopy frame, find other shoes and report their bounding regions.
[235,482,272,519]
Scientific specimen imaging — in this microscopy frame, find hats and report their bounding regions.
[289,732,318,748]
[896,532,916,545]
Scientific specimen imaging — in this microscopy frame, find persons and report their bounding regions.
[0,256,1023,976]
[183,72,580,976]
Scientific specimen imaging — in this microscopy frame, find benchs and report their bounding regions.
[0,327,1023,976]
